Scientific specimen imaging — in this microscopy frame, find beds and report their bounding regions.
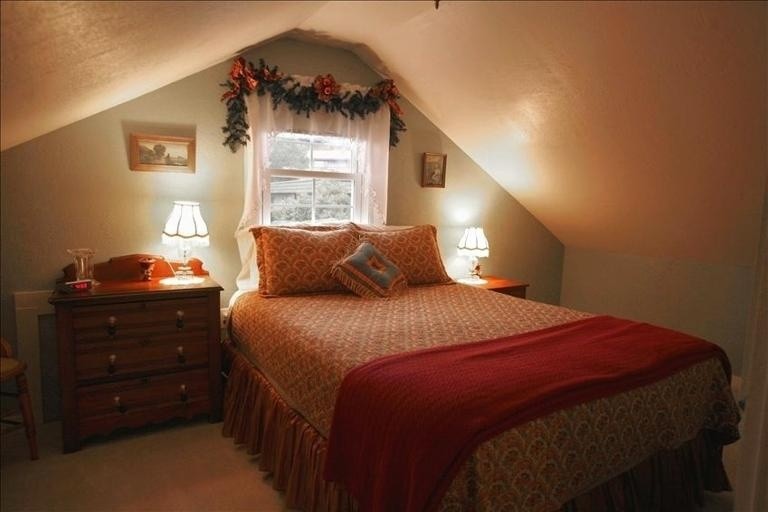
[220,282,741,512]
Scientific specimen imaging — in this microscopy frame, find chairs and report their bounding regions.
[0,338,39,460]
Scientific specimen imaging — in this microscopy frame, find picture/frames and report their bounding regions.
[421,152,446,187]
[130,132,196,176]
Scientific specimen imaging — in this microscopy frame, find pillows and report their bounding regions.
[330,235,408,300]
[357,224,457,287]
[249,224,359,298]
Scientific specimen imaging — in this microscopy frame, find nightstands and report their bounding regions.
[481,276,529,298]
[47,272,224,454]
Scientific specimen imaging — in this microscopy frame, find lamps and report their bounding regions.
[456,228,490,286]
[159,201,209,287]
